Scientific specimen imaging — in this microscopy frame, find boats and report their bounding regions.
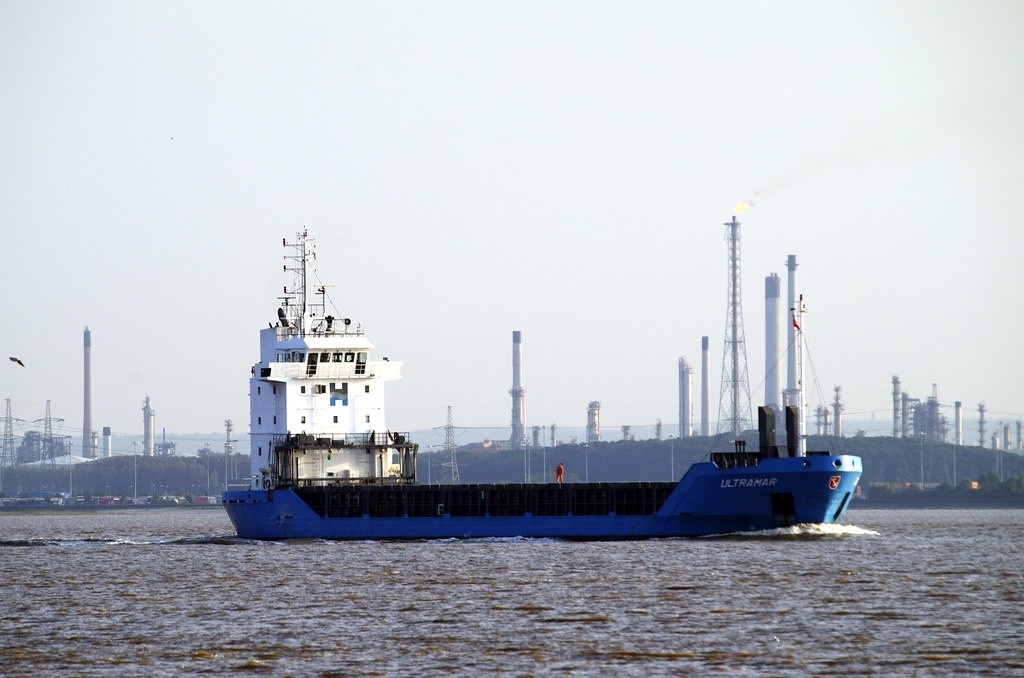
[220,222,864,542]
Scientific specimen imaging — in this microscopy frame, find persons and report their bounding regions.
[556,462,564,483]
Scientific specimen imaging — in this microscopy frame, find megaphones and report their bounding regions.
[342,318,351,325]
